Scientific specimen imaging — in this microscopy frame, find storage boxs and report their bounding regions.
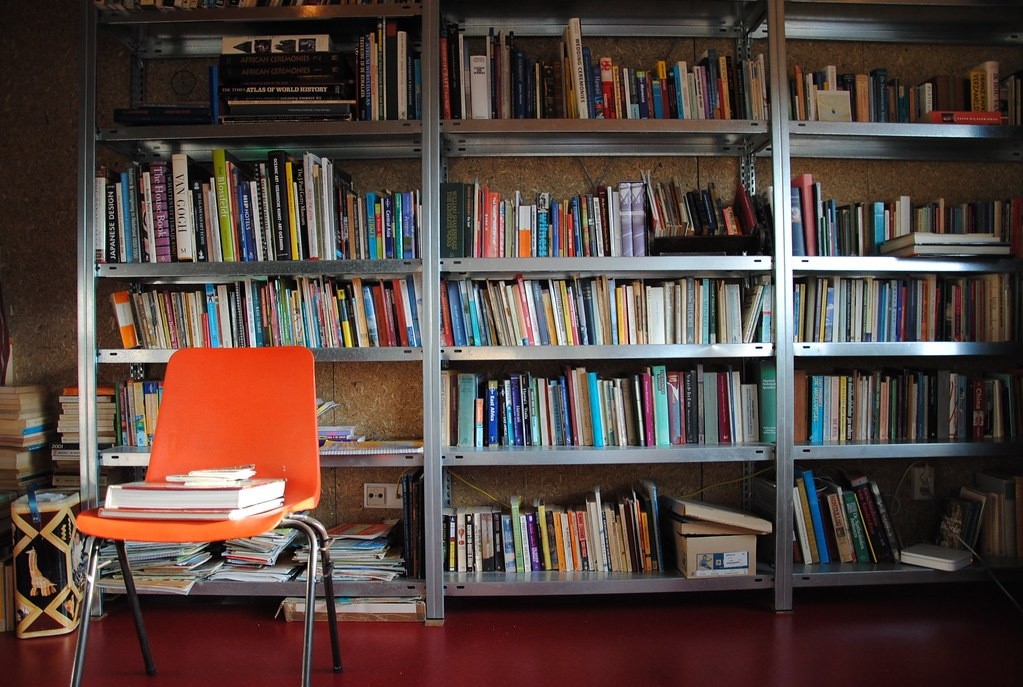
[673,520,758,579]
[274,596,427,624]
[668,492,772,535]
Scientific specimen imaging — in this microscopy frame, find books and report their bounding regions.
[788,60,1023,125]
[95,463,407,595]
[0,380,166,448]
[0,449,109,505]
[94,147,422,263]
[439,274,775,347]
[128,18,420,120]
[441,362,777,447]
[794,274,1019,343]
[793,463,1023,566]
[315,397,424,458]
[794,368,1023,444]
[442,479,664,574]
[792,172,1023,262]
[110,274,421,349]
[441,176,771,256]
[437,16,770,122]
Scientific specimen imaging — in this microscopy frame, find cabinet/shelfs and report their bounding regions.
[73,0,1023,628]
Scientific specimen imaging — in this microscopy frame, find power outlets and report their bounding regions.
[363,482,402,509]
[909,467,935,500]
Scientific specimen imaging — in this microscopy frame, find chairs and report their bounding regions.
[70,345,343,687]
